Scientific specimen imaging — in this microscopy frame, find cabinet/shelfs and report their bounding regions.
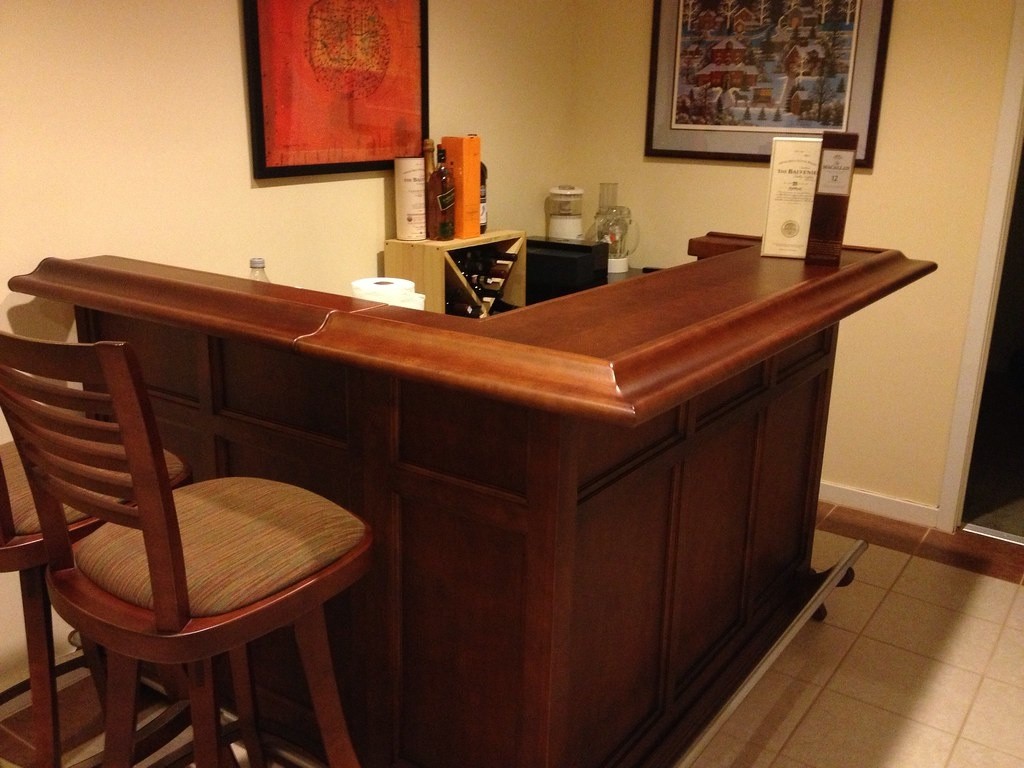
[384,230,527,318]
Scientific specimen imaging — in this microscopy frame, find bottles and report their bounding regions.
[466,134,488,235]
[246,257,269,282]
[422,139,437,186]
[446,243,518,319]
[426,148,455,240]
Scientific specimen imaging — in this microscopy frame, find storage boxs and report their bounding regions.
[441,136,481,238]
[760,137,823,258]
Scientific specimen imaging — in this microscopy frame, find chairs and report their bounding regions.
[0,333,373,768]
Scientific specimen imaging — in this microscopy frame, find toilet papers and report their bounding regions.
[351,277,426,311]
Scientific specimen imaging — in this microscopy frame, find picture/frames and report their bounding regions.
[242,0,431,180]
[642,0,893,169]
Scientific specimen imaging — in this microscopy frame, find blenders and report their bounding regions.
[548,185,586,242]
[594,183,640,273]
